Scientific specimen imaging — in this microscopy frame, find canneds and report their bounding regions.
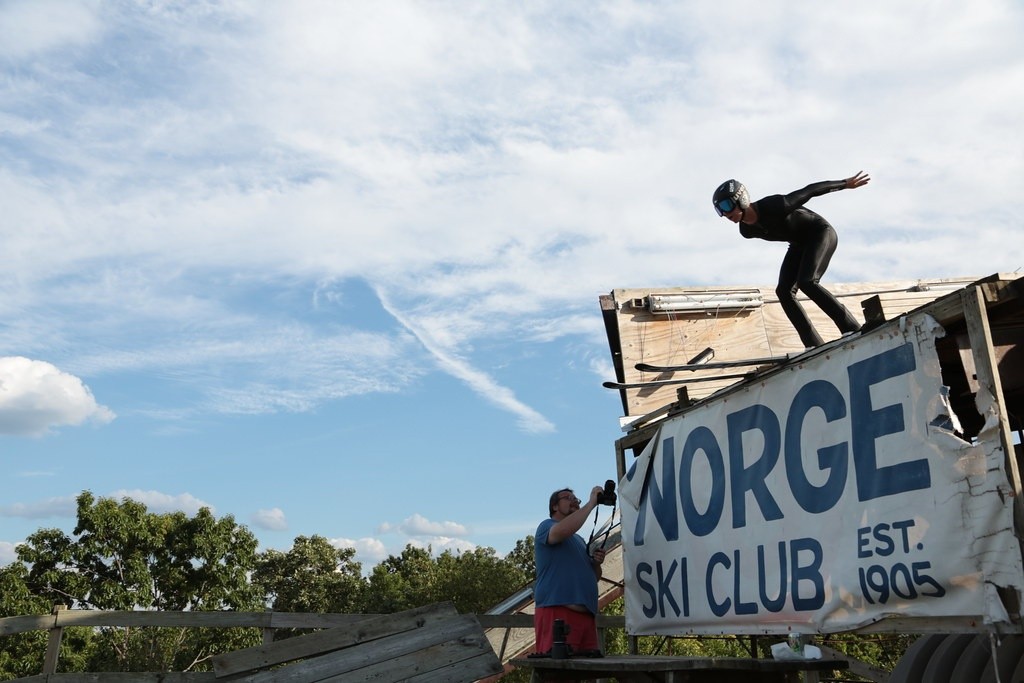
[788,631,805,658]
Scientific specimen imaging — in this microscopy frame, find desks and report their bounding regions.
[511,654,848,683]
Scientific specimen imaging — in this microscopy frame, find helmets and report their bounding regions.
[713,179,750,210]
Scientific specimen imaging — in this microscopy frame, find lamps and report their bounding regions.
[649,289,762,315]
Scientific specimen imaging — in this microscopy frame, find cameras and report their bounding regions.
[597,480,617,506]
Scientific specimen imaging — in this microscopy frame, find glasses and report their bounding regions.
[715,197,737,217]
[558,495,582,504]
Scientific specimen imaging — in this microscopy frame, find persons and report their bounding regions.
[534,485,606,683]
[712,170,871,353]
